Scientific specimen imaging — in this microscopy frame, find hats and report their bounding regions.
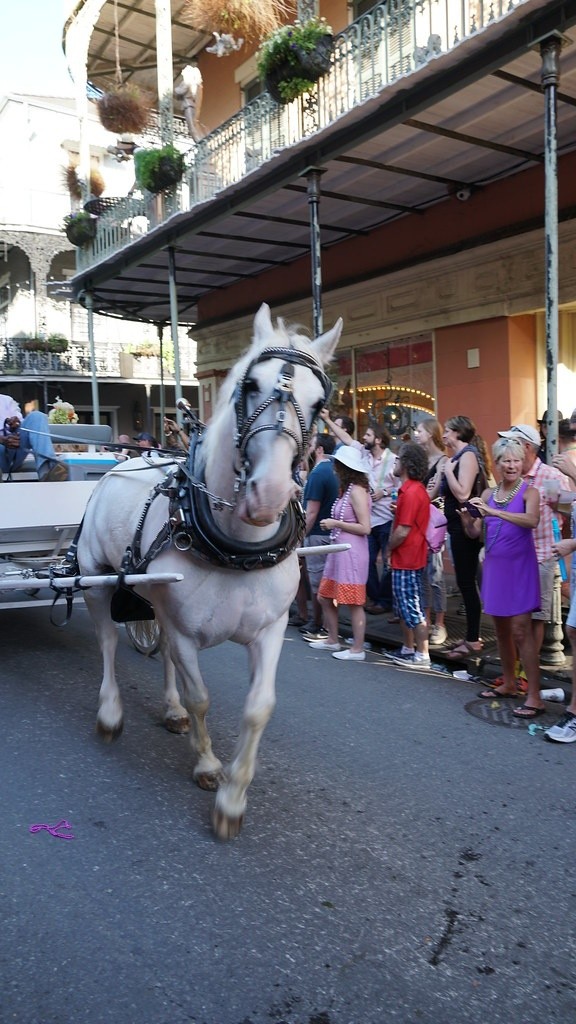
[132,432,155,443]
[119,435,130,444]
[537,409,563,424]
[323,446,370,474]
[496,424,542,446]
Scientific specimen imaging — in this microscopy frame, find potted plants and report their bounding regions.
[135,147,187,196]
[23,333,69,353]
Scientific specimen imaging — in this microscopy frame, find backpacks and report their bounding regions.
[406,485,448,554]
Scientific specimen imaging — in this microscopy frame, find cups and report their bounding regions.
[557,490,575,513]
[543,478,560,502]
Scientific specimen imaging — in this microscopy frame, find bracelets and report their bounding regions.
[176,428,183,434]
[165,431,172,437]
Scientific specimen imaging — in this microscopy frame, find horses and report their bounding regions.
[76,301,343,841]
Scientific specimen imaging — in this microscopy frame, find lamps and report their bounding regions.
[366,343,417,434]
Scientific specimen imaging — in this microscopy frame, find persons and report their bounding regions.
[288,408,576,742]
[54,416,190,459]
[0,394,69,481]
[174,65,205,144]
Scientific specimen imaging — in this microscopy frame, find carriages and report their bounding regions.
[0,301,344,841]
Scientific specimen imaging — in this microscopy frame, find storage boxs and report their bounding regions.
[59,454,118,482]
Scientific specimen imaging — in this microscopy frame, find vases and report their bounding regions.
[266,34,332,105]
[65,218,96,247]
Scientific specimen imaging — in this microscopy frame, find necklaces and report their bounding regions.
[329,483,352,540]
[300,459,331,506]
[493,477,524,506]
[378,450,390,488]
[530,462,543,487]
[561,447,576,453]
[482,480,519,552]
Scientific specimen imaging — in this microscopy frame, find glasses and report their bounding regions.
[395,456,401,460]
[510,426,533,442]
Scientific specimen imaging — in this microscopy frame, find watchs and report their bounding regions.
[383,489,388,496]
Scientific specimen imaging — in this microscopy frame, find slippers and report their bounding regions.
[513,704,545,718]
[477,689,519,699]
[444,639,486,649]
[445,641,482,661]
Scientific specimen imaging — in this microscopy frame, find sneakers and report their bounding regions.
[543,711,576,743]
[428,624,447,645]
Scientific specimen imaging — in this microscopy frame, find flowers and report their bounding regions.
[47,395,78,424]
[256,14,332,104]
[60,211,92,233]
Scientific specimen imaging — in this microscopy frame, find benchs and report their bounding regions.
[0,422,111,483]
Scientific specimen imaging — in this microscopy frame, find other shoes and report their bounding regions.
[298,620,320,633]
[361,601,393,615]
[308,641,340,651]
[331,649,366,660]
[302,633,330,641]
[386,616,404,623]
[39,463,68,482]
[299,627,331,636]
[287,614,309,626]
[383,648,401,659]
[393,652,431,669]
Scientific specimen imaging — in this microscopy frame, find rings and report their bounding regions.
[16,422,19,425]
[480,501,483,505]
[555,554,558,557]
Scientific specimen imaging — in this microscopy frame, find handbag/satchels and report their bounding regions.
[442,449,490,521]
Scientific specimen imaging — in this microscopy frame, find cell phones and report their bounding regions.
[464,501,482,519]
